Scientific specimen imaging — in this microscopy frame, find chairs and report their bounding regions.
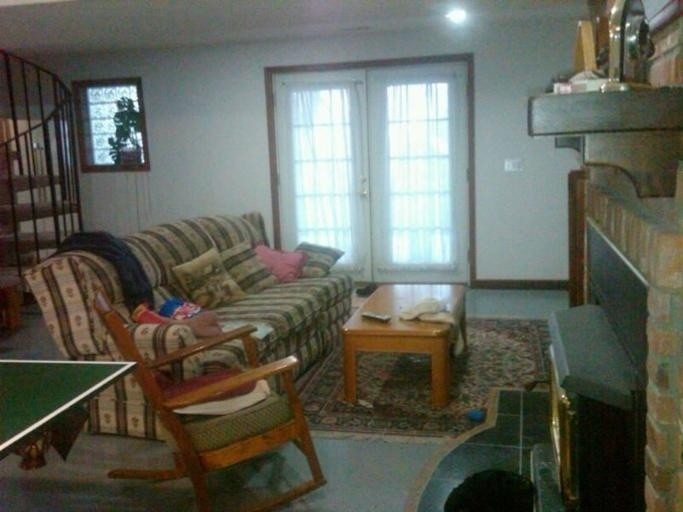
[92,289,329,509]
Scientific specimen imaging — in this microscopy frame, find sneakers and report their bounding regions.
[356,282,378,296]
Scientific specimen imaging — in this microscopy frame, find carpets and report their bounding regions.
[291,316,556,441]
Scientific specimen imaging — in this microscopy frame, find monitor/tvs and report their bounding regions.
[547,305,646,511]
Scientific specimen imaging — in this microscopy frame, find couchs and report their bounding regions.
[20,211,354,440]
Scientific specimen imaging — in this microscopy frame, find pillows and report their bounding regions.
[133,242,343,337]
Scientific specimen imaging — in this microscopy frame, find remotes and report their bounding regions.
[362,311,390,322]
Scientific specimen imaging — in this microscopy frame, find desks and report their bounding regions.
[1,360,138,454]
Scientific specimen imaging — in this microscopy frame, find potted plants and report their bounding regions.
[108,96,143,165]
